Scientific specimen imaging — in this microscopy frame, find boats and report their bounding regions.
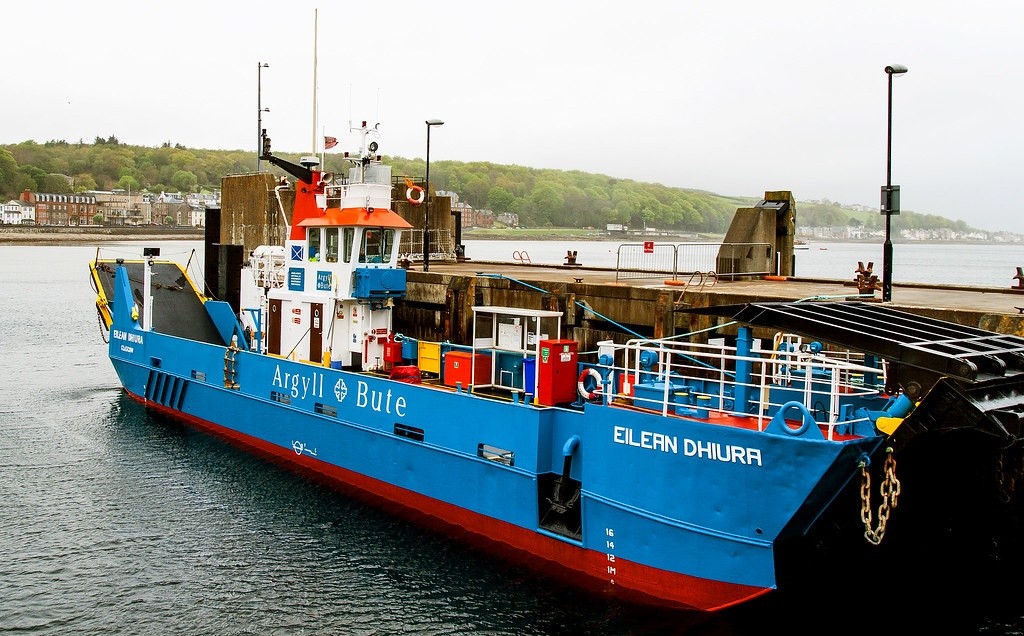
[88,4,1022,636]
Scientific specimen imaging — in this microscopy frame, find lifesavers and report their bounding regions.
[763,275,787,282]
[663,280,685,286]
[578,368,604,400]
[406,186,424,204]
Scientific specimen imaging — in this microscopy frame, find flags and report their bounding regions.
[324,136,339,149]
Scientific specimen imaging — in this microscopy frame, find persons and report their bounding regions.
[243,326,251,350]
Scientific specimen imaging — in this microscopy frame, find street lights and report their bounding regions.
[882,65,908,302]
[423,118,445,273]
[257,62,270,171]
